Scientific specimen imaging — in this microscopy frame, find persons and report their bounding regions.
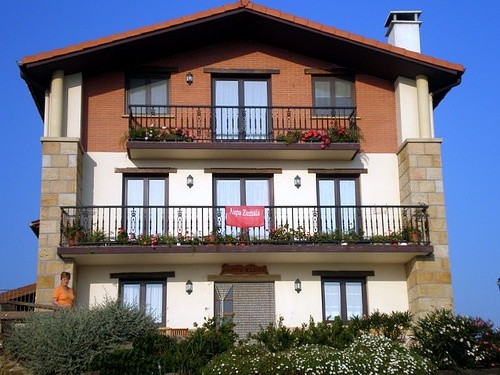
[53,272,75,311]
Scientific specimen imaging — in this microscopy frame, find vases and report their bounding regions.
[67,235,78,246]
[204,235,248,247]
[390,237,398,245]
[410,230,420,245]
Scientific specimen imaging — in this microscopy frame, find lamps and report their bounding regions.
[185,280,192,294]
[187,175,193,188]
[294,175,301,188]
[186,72,193,85]
[294,279,301,293]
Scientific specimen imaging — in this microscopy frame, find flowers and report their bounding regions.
[275,120,361,149]
[63,203,430,245]
[127,121,199,142]
